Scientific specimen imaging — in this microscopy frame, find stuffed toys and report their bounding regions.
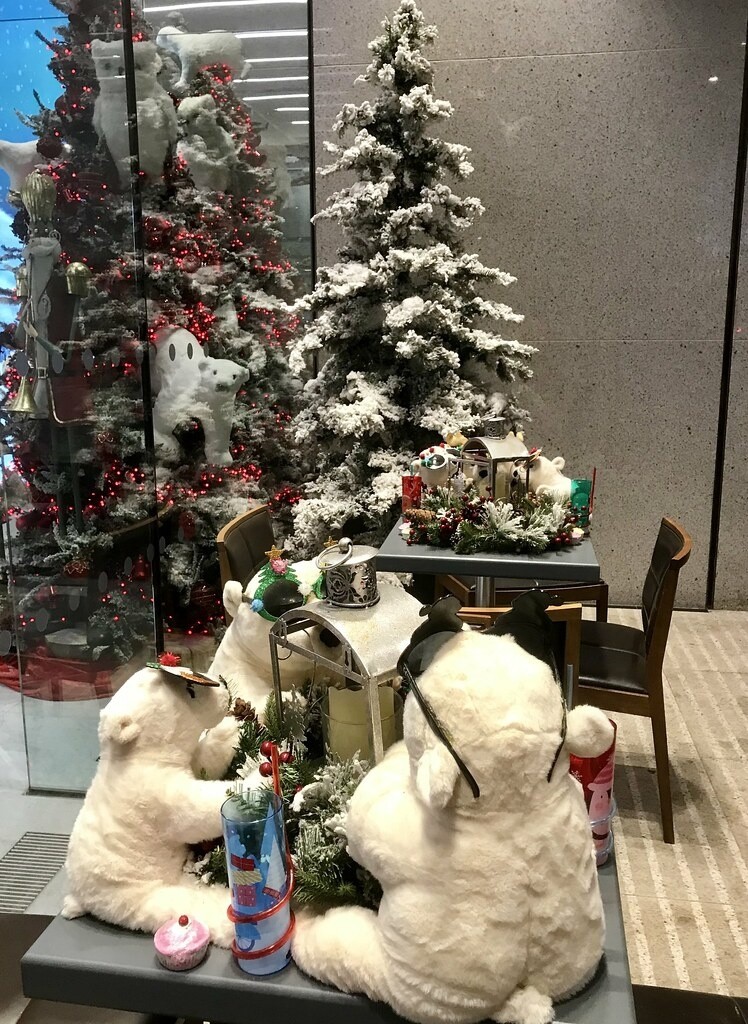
[291,630,614,1024]
[206,556,347,744]
[63,656,306,942]
[514,454,571,501]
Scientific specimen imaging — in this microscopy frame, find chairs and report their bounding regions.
[568,514,693,844]
[434,571,611,622]
[458,605,583,714]
[215,504,277,626]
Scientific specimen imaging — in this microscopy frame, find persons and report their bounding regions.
[23,252,93,534]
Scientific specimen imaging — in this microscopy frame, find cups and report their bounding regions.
[481,414,506,440]
[401,476,422,522]
[571,480,590,538]
[222,791,292,975]
[570,718,618,866]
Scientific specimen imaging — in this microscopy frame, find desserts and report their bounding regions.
[153,915,209,971]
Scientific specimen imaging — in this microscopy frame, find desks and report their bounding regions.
[22,829,637,1024]
[372,511,601,608]
